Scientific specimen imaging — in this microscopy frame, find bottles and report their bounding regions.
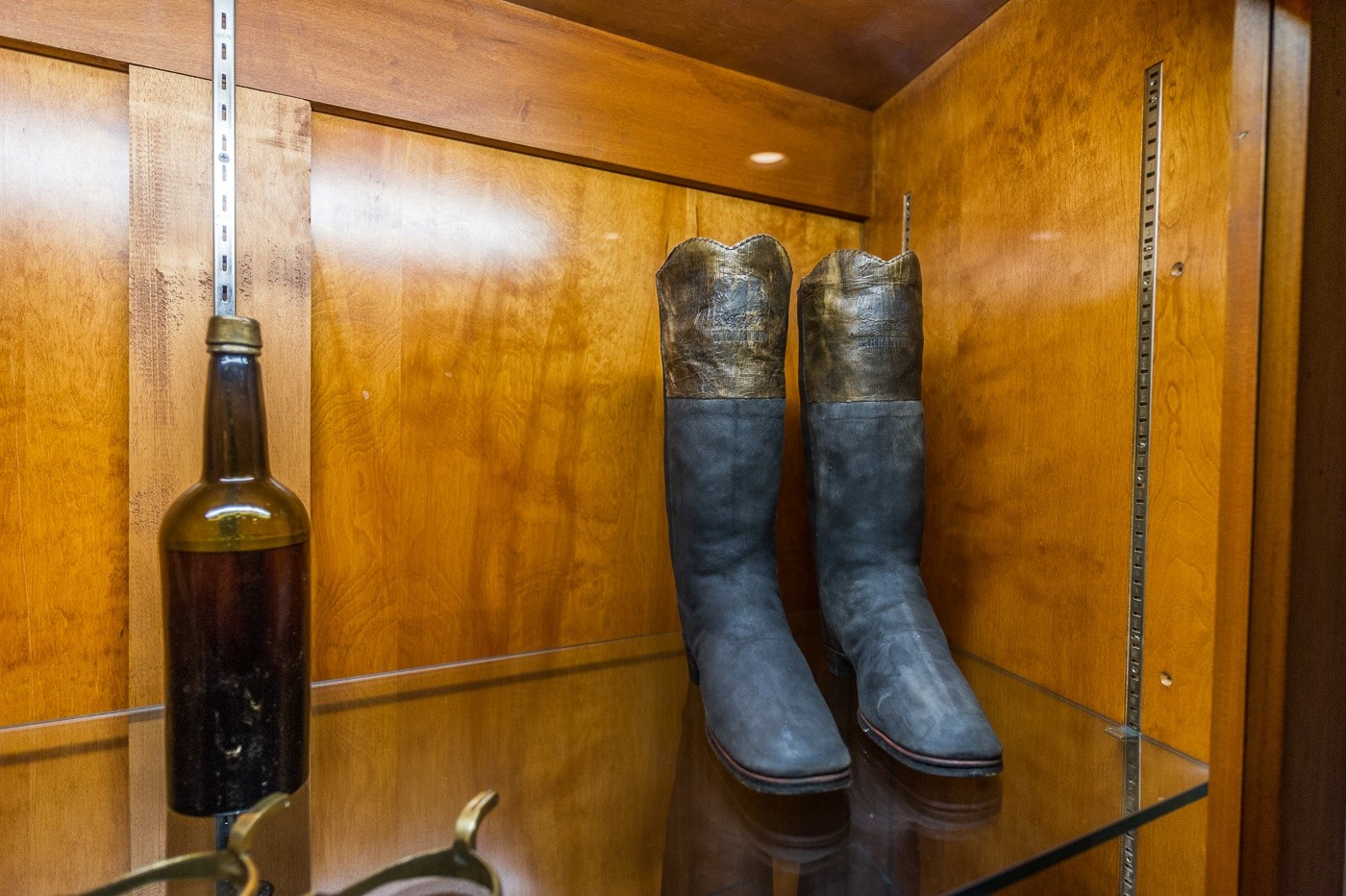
[160,314,313,817]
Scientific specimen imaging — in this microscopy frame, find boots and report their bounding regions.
[798,248,1006,778]
[653,235,853,793]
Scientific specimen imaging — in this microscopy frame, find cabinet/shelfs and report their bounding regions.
[2,0,1279,895]
